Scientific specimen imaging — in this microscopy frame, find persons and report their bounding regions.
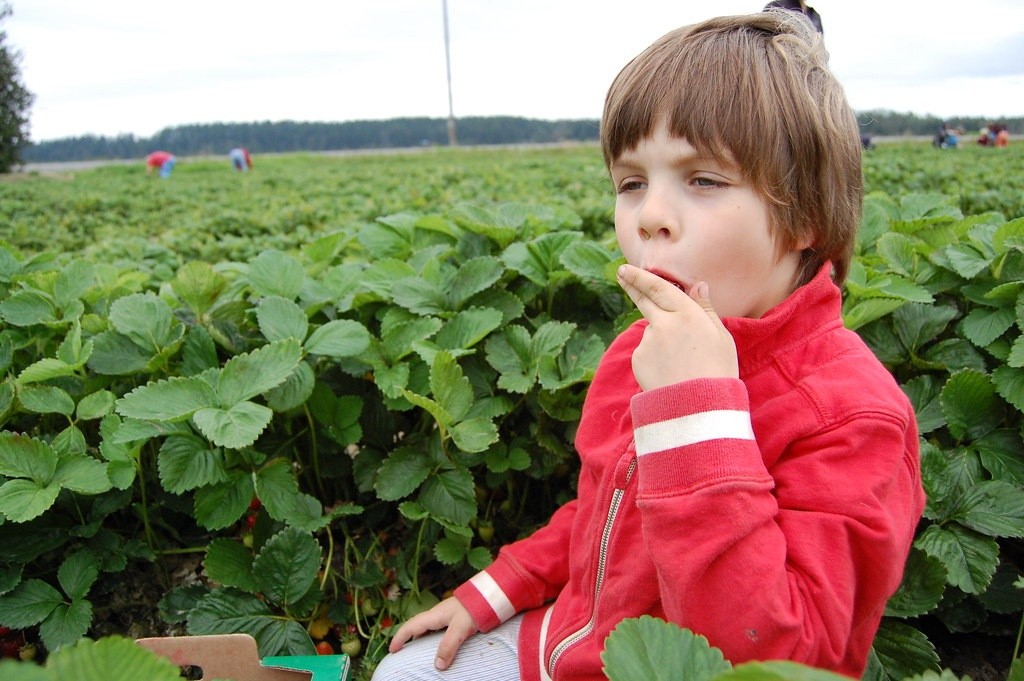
[229,147,253,173]
[977,121,1009,148]
[932,121,967,150]
[145,151,176,180]
[368,0,928,680]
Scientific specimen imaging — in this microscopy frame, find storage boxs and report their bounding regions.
[136,634,350,681]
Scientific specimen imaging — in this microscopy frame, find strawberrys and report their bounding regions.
[248,496,395,655]
